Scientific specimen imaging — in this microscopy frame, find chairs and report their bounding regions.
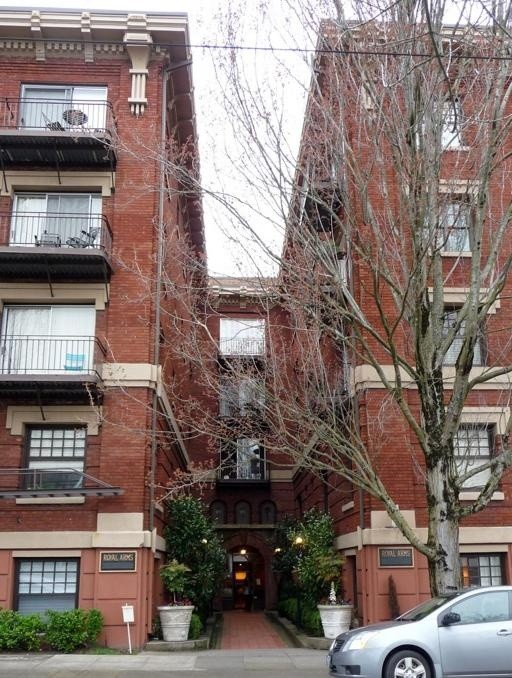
[36,231,61,247]
[43,113,64,131]
[65,224,101,249]
[64,353,86,371]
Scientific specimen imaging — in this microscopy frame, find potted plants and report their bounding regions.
[156,559,197,642]
[316,556,353,639]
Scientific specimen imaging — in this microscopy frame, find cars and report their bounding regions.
[323,584,512,678]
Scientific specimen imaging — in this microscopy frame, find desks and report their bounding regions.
[63,110,89,130]
